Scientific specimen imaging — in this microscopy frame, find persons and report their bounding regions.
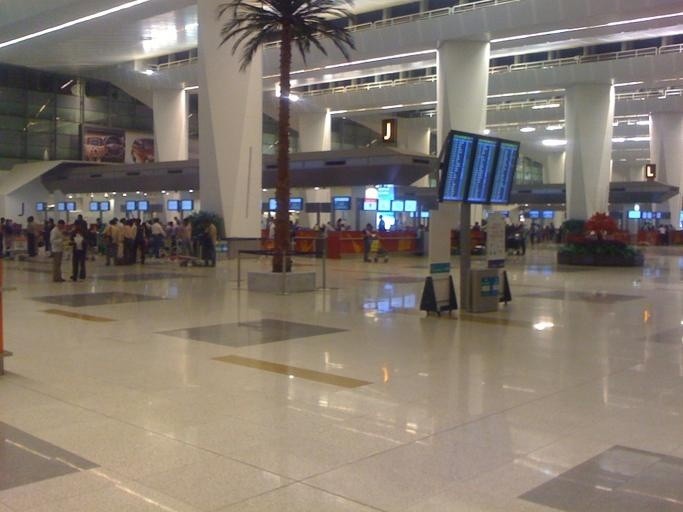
[262,214,386,235]
[638,224,673,246]
[49,219,66,283]
[69,220,86,282]
[0,212,219,267]
[362,222,375,263]
[475,219,557,255]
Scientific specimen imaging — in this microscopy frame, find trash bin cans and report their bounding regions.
[471,267,499,313]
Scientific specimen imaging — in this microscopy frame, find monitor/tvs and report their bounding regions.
[99,202,110,211]
[526,207,663,220]
[88,202,99,211]
[57,202,66,211]
[181,200,193,211]
[137,200,148,211]
[268,195,417,212]
[35,201,45,211]
[125,200,136,211]
[65,202,76,211]
[167,200,179,210]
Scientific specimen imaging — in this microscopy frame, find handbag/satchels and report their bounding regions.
[102,236,112,246]
